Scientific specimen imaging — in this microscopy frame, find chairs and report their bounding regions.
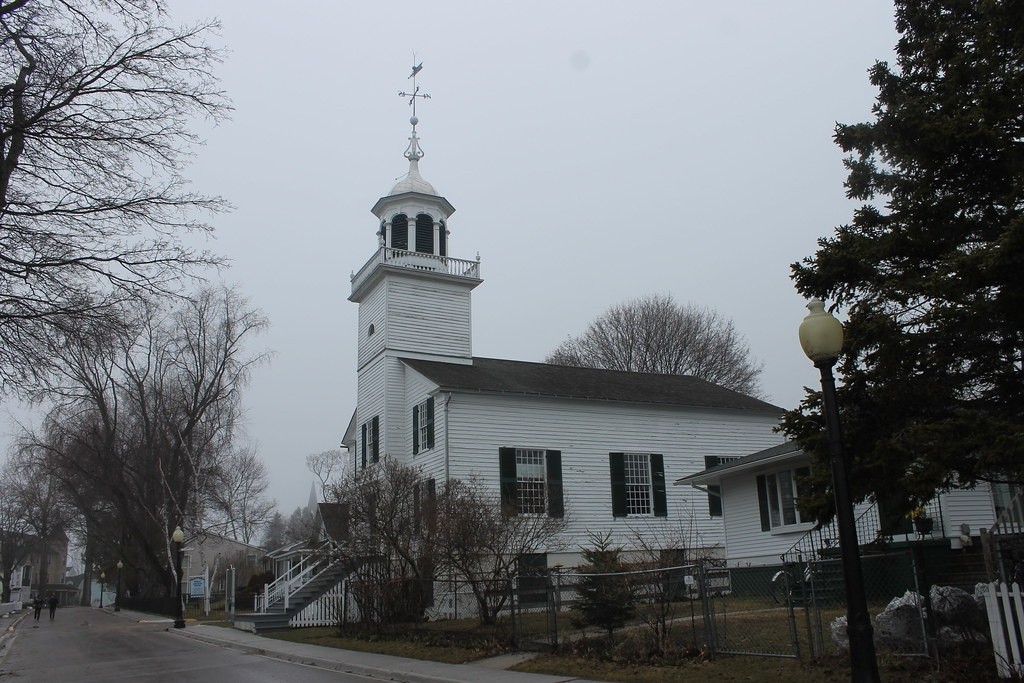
[915,517,935,540]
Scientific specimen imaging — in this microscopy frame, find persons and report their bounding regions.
[48,592,59,622]
[33,595,42,622]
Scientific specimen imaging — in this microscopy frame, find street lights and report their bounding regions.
[173,526,186,629]
[98,571,106,607]
[798,295,881,683]
